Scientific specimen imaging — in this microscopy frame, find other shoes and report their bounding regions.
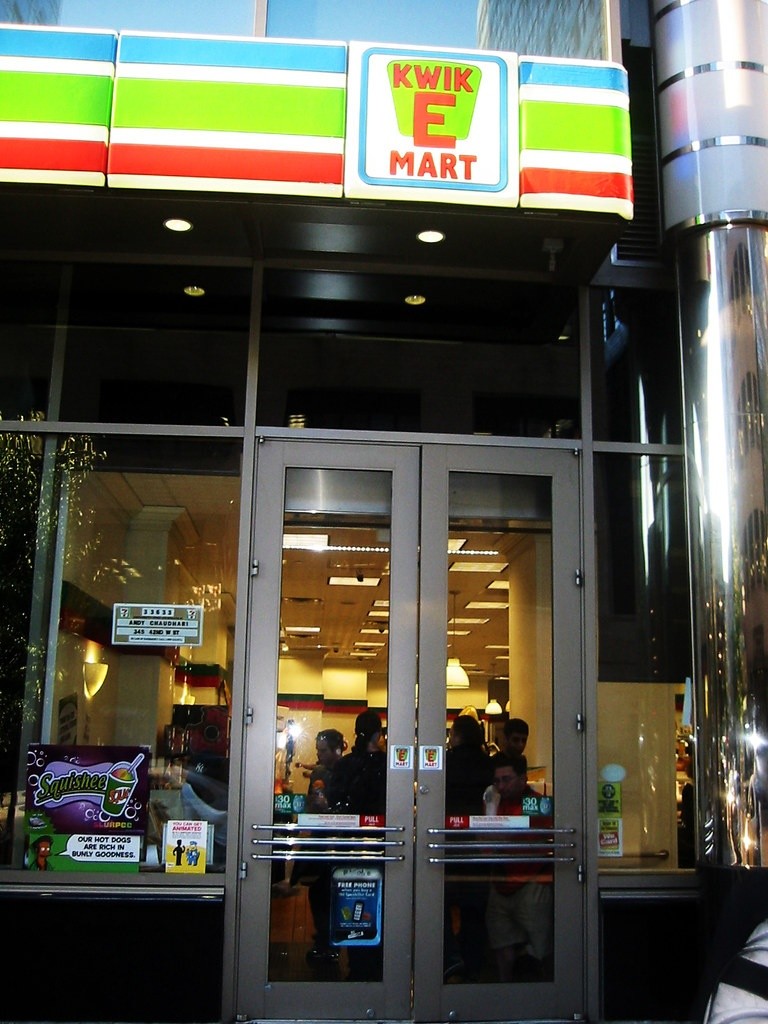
[443,960,462,983]
[328,948,340,962]
[307,945,324,960]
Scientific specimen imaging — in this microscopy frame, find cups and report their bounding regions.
[485,792,501,815]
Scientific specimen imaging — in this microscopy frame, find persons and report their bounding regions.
[153,712,555,982]
[680,763,695,834]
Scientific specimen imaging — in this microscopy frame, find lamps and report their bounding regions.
[485,663,503,716]
[446,592,469,691]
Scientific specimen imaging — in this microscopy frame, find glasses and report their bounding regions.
[492,775,520,786]
